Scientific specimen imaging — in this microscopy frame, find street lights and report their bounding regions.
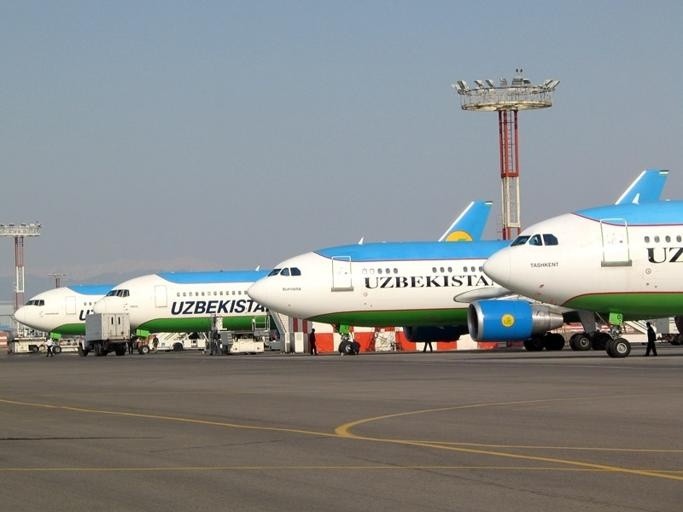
[0,223,41,311]
[451,77,560,240]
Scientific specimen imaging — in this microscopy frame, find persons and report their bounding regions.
[127,342,133,354]
[310,329,318,355]
[643,322,657,356]
[153,335,159,354]
[210,329,220,355]
[46,338,54,357]
